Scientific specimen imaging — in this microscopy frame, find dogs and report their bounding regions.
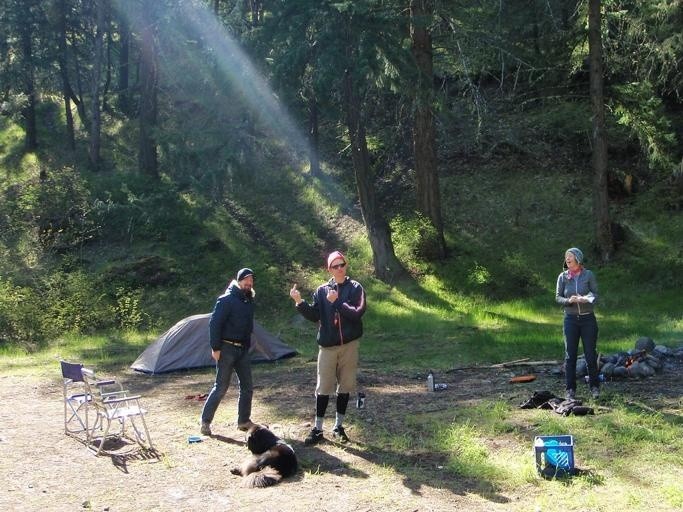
[229,423,298,489]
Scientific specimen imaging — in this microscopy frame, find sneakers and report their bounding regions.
[200,422,211,434]
[237,421,255,430]
[332,426,348,443]
[590,387,600,399]
[564,388,575,399]
[304,427,323,445]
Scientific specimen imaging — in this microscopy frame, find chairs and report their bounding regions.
[59,359,162,464]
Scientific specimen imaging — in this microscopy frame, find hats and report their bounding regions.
[327,251,345,270]
[562,247,584,271]
[236,267,256,281]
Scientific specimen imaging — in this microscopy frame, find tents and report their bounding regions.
[130,313,297,376]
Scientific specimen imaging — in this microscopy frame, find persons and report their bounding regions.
[201,268,257,434]
[554,247,600,399]
[289,251,365,444]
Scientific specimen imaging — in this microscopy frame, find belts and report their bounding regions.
[223,340,242,346]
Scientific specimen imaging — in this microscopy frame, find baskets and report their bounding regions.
[535,435,574,478]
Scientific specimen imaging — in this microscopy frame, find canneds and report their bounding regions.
[434,383,447,389]
[356,397,365,408]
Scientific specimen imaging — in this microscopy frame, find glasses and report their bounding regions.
[330,259,345,269]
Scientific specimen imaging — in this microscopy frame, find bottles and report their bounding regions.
[426,374,434,392]
[356,392,365,409]
